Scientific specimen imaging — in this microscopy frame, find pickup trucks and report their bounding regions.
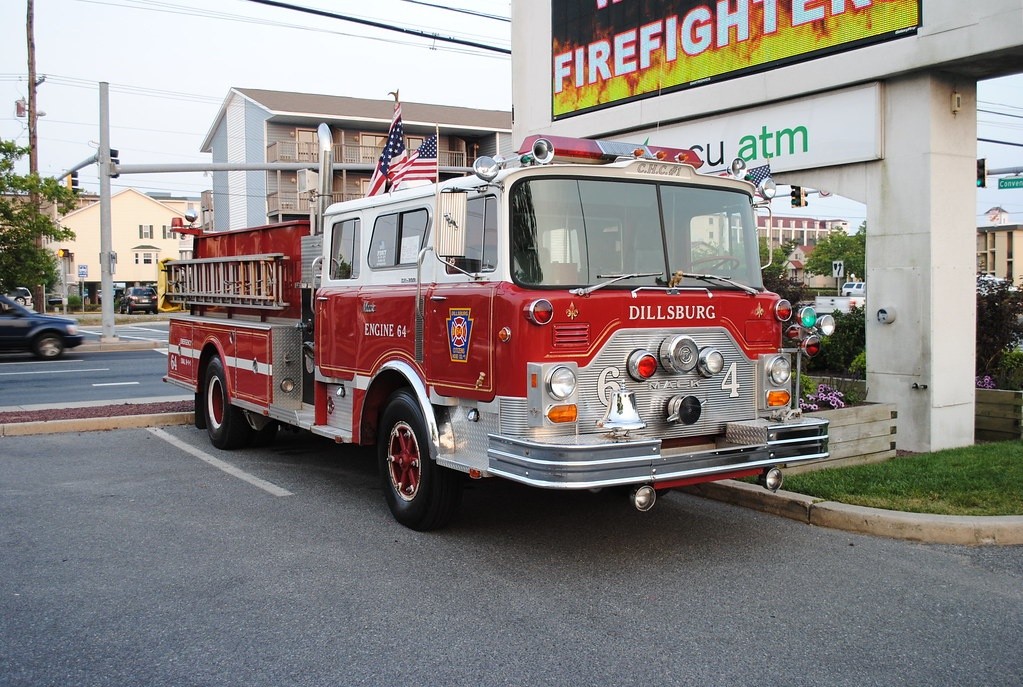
[812,295,865,317]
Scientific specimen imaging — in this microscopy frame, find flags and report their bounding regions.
[388,136,437,193]
[365,103,407,197]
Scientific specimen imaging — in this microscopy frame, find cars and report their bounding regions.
[48,289,102,306]
[0,294,83,358]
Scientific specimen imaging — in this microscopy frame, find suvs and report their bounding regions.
[841,281,866,295]
[119,286,159,316]
[1,288,34,311]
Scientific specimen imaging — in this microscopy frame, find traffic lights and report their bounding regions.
[976,158,986,188]
[787,184,806,209]
[66,172,80,194]
[59,249,69,258]
[109,148,121,178]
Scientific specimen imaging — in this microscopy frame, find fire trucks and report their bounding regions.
[160,122,836,531]
